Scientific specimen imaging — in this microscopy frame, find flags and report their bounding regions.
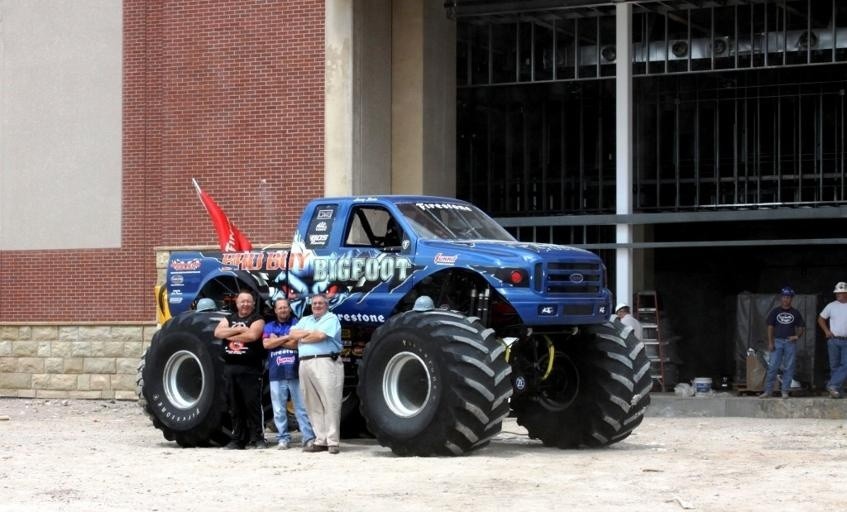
[199,188,251,252]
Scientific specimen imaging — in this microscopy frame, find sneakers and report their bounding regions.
[277,440,288,450]
[302,439,339,453]
[225,439,266,449]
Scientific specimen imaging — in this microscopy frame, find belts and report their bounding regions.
[299,353,331,360]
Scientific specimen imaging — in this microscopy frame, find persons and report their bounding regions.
[615,303,644,345]
[215,290,266,449]
[290,294,345,454]
[262,298,315,450]
[817,282,847,399]
[759,286,805,399]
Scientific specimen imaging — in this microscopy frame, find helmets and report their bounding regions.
[413,295,434,312]
[833,282,847,293]
[781,287,794,296]
[195,298,217,312]
[615,304,630,312]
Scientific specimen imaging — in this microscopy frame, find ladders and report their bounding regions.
[636,290,665,392]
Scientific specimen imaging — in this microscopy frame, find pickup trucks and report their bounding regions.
[135,176,654,458]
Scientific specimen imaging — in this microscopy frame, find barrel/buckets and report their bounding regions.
[692,377,713,397]
